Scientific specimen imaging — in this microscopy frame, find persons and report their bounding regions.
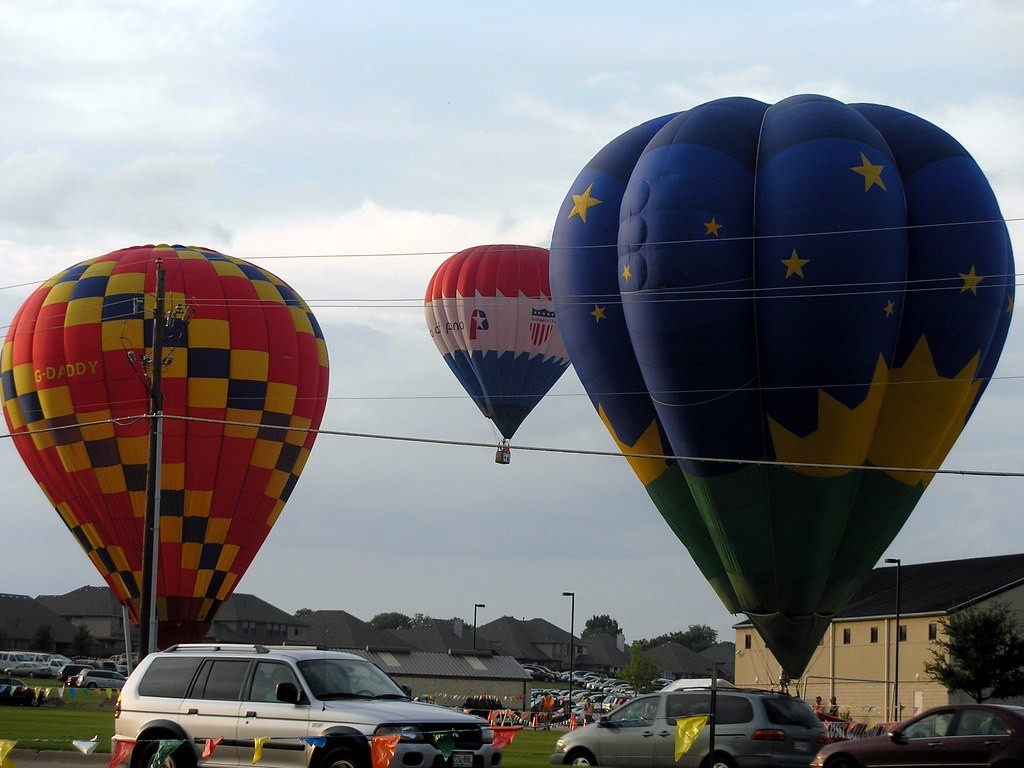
[539,691,555,730]
[584,699,595,726]
[828,696,839,717]
[812,696,826,714]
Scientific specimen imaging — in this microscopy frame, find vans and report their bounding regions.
[0,652,30,673]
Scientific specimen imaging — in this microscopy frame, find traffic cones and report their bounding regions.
[532,714,538,728]
[583,719,587,727]
[569,715,577,730]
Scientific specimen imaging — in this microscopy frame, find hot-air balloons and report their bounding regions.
[0,244,330,647]
[424,243,572,467]
[549,92,1015,679]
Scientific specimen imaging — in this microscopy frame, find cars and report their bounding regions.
[30,653,140,676]
[651,678,673,688]
[68,669,82,687]
[658,679,738,692]
[521,662,645,719]
[809,705,1024,768]
[4,662,52,678]
[0,679,47,708]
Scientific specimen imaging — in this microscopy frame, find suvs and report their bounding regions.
[77,669,128,690]
[57,664,96,683]
[111,644,503,768]
[550,687,828,768]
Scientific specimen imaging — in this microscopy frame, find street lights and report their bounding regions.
[884,557,901,721]
[562,592,574,718]
[473,603,486,650]
